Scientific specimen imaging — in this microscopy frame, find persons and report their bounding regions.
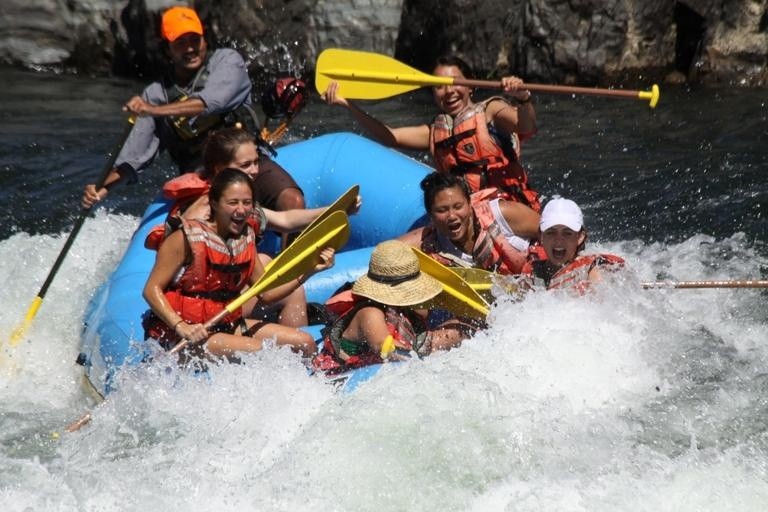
[141,168,317,370]
[396,169,541,276]
[511,195,632,299]
[79,6,306,255]
[320,52,549,214]
[300,238,480,377]
[158,126,364,327]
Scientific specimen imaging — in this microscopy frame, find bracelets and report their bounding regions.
[173,320,185,337]
[513,90,531,105]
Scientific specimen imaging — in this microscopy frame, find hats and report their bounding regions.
[540,198,583,232]
[161,6,203,42]
[350,240,443,306]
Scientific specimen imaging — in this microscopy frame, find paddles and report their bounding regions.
[64,211,349,431]
[249,185,361,289]
[411,247,493,322]
[9,111,136,345]
[316,47,660,108]
[449,268,768,302]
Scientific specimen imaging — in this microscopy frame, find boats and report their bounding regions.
[74,130,510,419]
